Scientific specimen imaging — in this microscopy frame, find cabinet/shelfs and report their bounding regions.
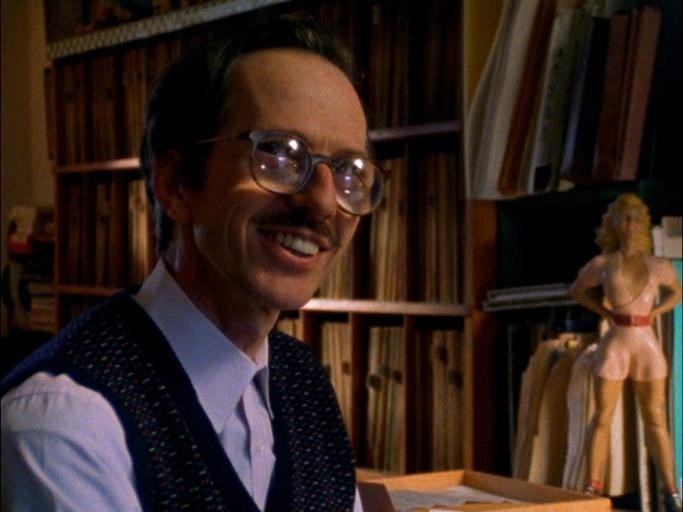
[46,1,503,476]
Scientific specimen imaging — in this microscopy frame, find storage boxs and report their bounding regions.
[354,470,610,512]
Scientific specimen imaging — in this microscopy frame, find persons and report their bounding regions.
[571,192,683,511]
[0,14,387,512]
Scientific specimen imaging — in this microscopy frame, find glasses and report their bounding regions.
[180,124,389,219]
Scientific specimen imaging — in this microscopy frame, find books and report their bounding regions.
[45,1,464,167]
[465,2,661,200]
[69,153,466,304]
[275,318,468,476]
[495,258,681,496]
